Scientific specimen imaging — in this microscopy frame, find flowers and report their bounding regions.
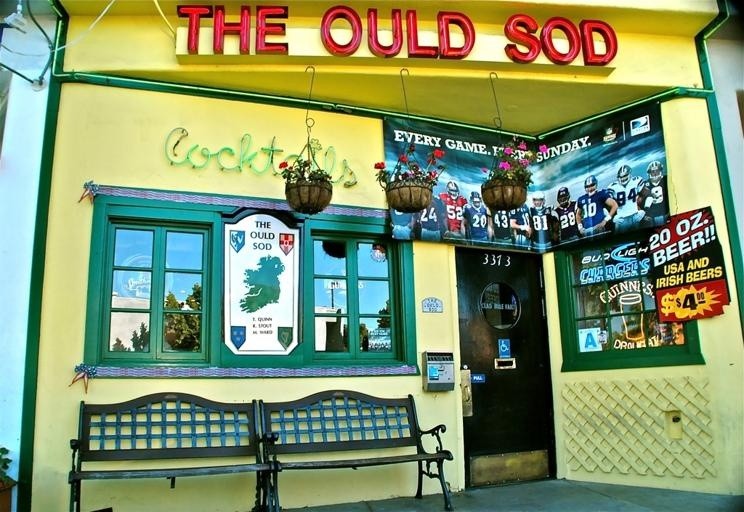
[374,143,448,185]
[482,143,547,180]
[279,160,329,182]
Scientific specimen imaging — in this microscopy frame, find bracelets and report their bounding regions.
[519,224,526,230]
[603,214,611,222]
[578,223,583,231]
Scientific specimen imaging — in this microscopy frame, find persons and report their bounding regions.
[388,206,419,239]
[440,181,467,238]
[459,192,493,243]
[607,164,651,234]
[529,191,555,252]
[487,208,516,246]
[575,175,618,236]
[509,203,533,250]
[553,188,579,244]
[637,160,669,224]
[416,192,446,240]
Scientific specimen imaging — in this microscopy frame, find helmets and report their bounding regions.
[447,181,483,202]
[531,160,663,201]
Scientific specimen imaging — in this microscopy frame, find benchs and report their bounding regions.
[259,390,453,512]
[68,392,272,512]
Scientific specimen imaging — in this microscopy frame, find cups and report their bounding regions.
[619,294,650,342]
